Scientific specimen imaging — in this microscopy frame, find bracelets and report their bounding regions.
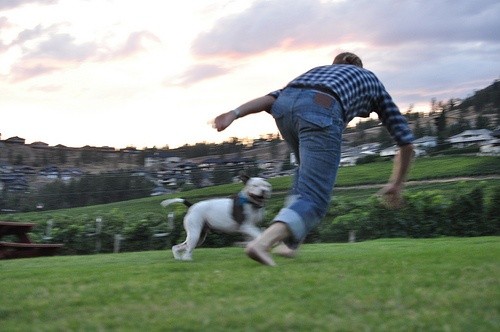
[232,109,240,118]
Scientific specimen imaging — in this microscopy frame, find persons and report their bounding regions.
[209,52,413,266]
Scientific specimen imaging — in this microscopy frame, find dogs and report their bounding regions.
[159,166,280,266]
